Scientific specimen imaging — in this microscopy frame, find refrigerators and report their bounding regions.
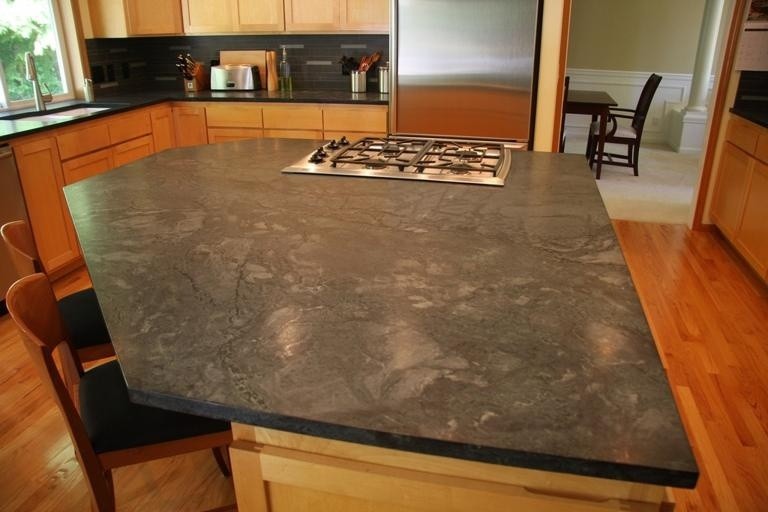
[388,0,539,153]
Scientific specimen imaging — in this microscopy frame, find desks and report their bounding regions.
[566,87,620,180]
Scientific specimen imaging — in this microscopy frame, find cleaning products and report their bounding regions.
[279,46,294,92]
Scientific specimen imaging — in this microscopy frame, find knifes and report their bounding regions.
[176,52,195,80]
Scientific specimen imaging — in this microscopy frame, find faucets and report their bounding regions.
[24,50,54,112]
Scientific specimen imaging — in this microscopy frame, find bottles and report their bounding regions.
[377,65,388,95]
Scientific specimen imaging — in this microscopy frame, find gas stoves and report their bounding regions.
[282,134,512,188]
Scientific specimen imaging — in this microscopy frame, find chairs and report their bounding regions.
[585,71,664,181]
[1,220,119,464]
[1,272,235,511]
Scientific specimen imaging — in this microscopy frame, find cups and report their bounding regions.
[350,70,368,93]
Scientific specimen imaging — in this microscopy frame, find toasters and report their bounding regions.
[209,64,261,93]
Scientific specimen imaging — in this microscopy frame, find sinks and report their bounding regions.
[2,112,79,122]
[49,102,130,117]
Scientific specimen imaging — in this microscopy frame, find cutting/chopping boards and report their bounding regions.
[218,49,267,90]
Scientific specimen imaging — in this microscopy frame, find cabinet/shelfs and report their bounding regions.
[705,117,768,283]
[56,107,156,186]
[207,103,389,144]
[149,106,175,153]
[180,1,393,33]
[9,136,83,285]
[86,0,182,40]
[170,104,209,150]
